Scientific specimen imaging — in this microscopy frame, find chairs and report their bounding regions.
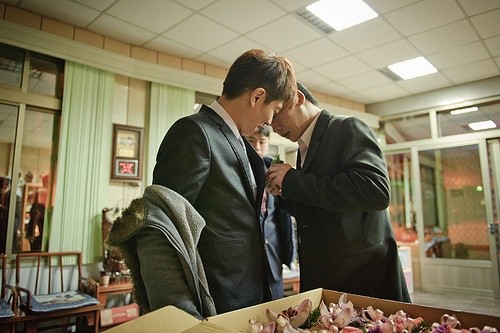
[0,252,99,333]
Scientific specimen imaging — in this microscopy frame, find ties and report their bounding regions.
[238,135,257,200]
[260,189,267,218]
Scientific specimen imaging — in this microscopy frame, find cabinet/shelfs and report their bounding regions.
[16,183,43,252]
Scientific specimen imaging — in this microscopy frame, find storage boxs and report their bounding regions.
[101,288,500,333]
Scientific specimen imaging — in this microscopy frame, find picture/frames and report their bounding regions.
[111,124,143,180]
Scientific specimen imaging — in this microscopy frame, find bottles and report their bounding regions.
[271,154,284,165]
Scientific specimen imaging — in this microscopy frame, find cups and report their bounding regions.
[100,271,110,285]
[111,270,131,285]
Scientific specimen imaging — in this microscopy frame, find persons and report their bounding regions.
[138,48,299,321]
[243,126,293,299]
[0,177,47,254]
[265,82,412,304]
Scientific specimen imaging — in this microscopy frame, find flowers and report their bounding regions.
[247,293,499,333]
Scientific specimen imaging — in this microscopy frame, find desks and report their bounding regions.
[83,281,138,309]
